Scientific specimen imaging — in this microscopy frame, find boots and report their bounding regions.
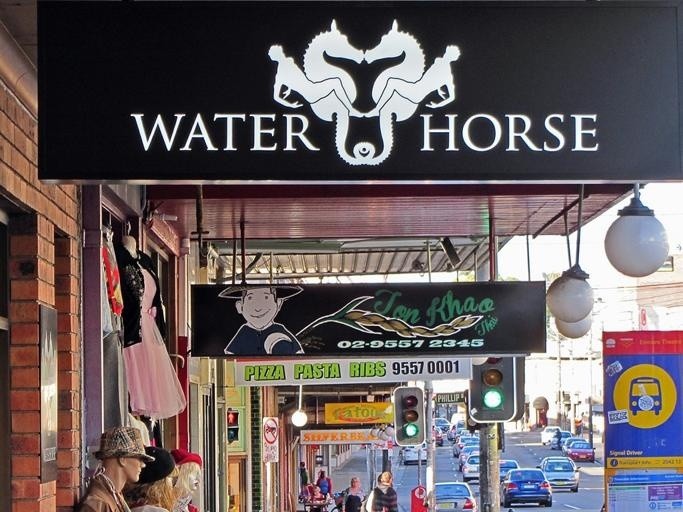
[401,443,429,464]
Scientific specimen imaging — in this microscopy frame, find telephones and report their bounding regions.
[436,392,468,406]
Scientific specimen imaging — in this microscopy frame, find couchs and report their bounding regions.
[225,410,239,442]
[394,388,427,447]
[466,356,518,421]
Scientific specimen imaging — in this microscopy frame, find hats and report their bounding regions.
[433,427,445,447]
[434,417,450,432]
[434,482,478,512]
[551,432,574,450]
[447,416,553,512]
[539,426,561,446]
[536,456,582,493]
[567,442,596,463]
[561,438,587,458]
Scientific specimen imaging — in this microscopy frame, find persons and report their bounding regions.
[102,225,128,425]
[299,461,308,497]
[341,476,368,512]
[124,445,174,512]
[171,446,202,511]
[553,428,562,449]
[315,469,332,500]
[115,237,188,418]
[365,471,398,512]
[73,426,155,511]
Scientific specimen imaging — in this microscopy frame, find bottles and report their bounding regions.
[93,428,202,482]
[219,286,303,301]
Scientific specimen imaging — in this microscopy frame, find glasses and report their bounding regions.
[291,379,308,428]
[546,184,669,339]
